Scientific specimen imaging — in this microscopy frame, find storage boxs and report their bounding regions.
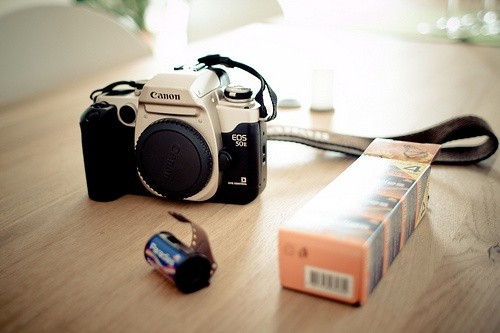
[277,137,441,308]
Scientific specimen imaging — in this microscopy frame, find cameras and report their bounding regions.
[79,63,268,204]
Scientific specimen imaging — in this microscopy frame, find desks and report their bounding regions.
[0,23,500,333]
